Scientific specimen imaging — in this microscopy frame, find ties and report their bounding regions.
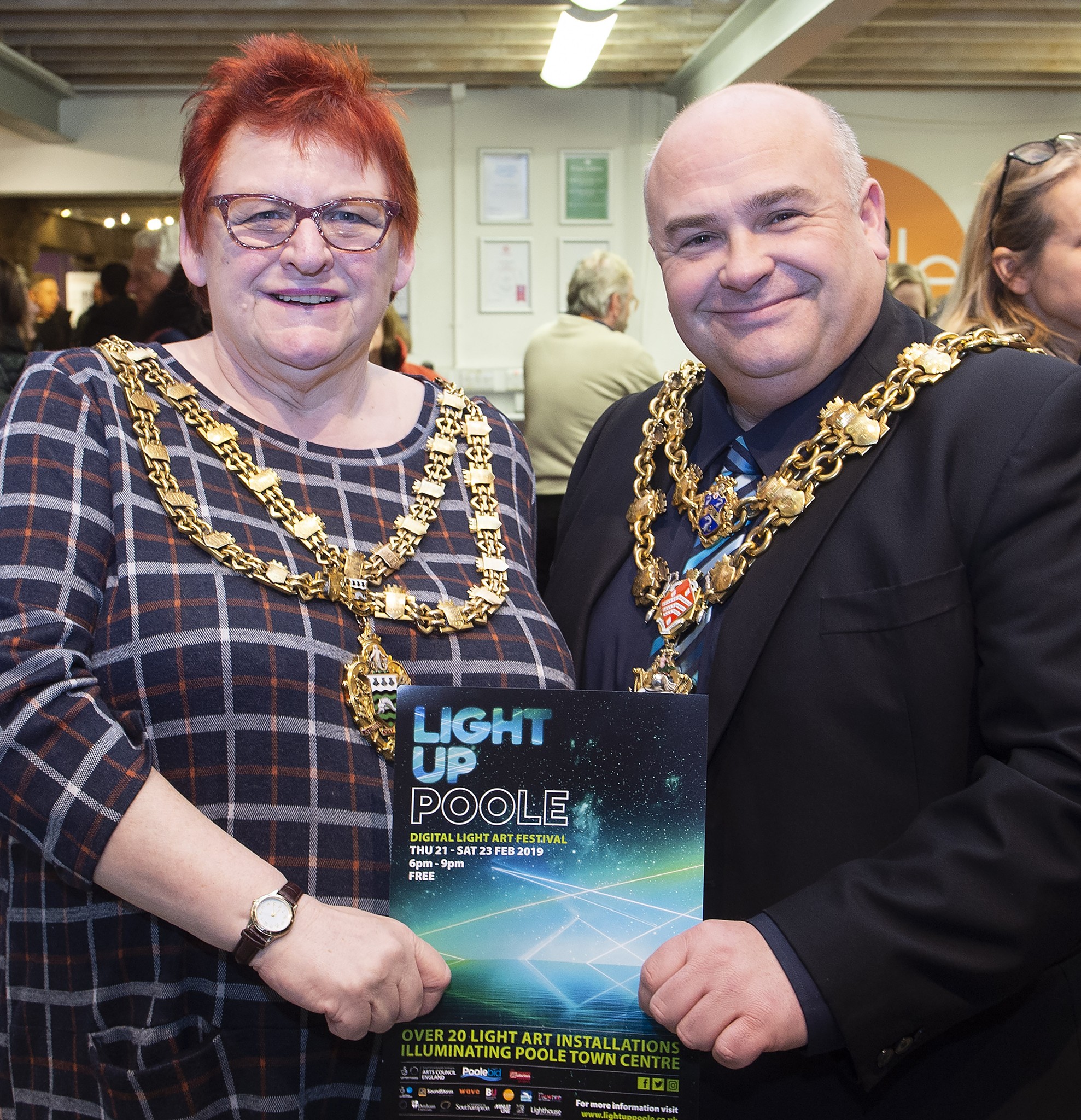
[652,434,763,696]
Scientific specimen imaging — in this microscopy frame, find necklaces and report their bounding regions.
[95,334,510,763]
[625,328,1047,694]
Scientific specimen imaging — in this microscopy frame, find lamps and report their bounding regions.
[539,1,626,89]
[669,0,833,105]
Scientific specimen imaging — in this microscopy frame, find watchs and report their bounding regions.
[231,880,303,968]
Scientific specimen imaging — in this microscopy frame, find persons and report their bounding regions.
[1,31,1080,1120]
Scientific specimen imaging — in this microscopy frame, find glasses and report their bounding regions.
[987,129,1081,249]
[203,191,403,254]
